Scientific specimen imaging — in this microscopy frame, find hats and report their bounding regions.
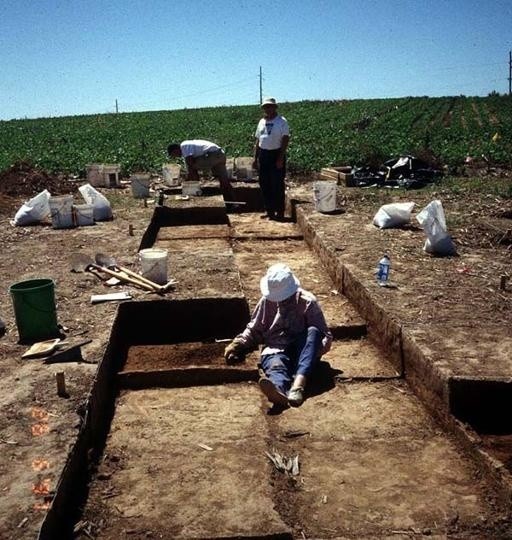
[261,97,278,109]
[166,144,178,157]
[259,263,300,302]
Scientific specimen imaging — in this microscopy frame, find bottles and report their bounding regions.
[377,255,391,287]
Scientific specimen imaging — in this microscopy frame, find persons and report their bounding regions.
[166,138,233,201]
[251,97,292,221]
[224,264,333,406]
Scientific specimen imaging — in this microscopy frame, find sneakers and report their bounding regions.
[288,383,305,406]
[260,212,285,221]
[259,377,289,409]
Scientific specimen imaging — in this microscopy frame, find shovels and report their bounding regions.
[70,253,164,290]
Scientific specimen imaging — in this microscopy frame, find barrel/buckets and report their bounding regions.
[9,279,60,341]
[180,181,200,196]
[224,158,234,178]
[47,195,74,229]
[140,248,169,284]
[163,165,181,186]
[74,204,94,226]
[314,179,337,213]
[130,174,150,198]
[235,156,253,181]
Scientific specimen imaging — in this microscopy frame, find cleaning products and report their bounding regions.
[377,253,391,282]
